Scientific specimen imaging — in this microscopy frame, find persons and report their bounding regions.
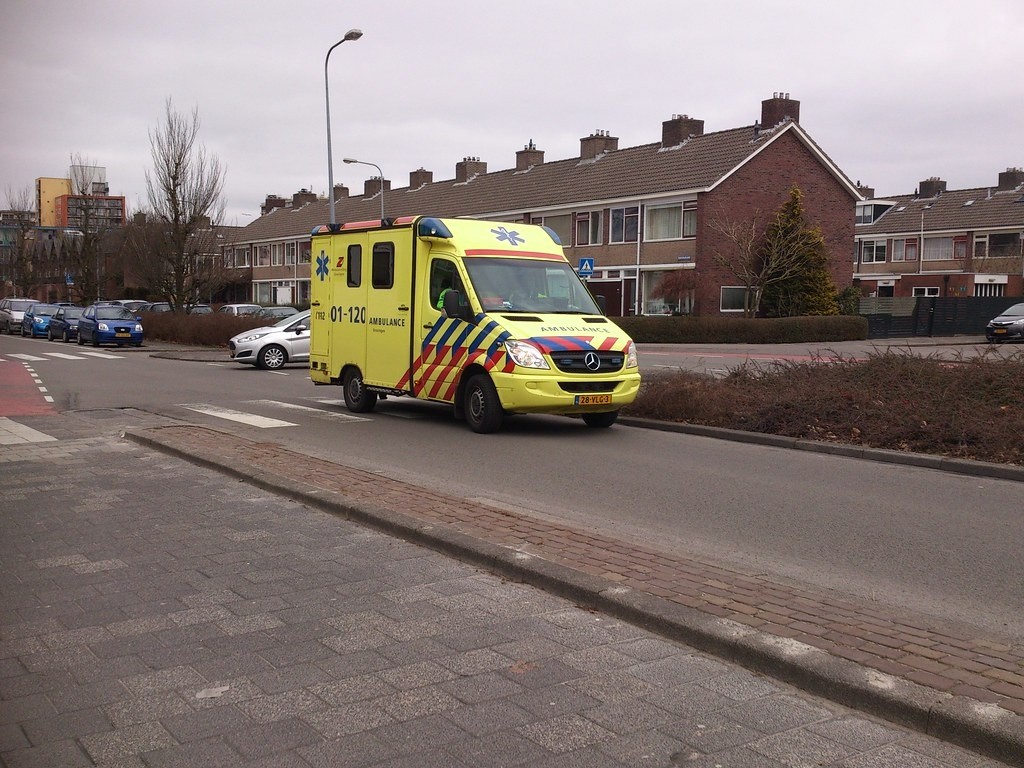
[508,271,548,303]
[437,269,483,308]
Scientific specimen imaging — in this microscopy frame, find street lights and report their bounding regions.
[342,158,385,220]
[325,29,363,224]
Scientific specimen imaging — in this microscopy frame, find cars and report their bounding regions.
[21,304,60,339]
[48,307,86,344]
[76,305,143,348]
[228,309,311,371]
[252,306,300,317]
[985,303,1024,344]
[109,300,148,313]
[134,302,175,312]
[217,304,264,316]
[183,304,214,315]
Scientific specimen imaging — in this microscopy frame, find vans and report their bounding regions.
[0,299,40,336]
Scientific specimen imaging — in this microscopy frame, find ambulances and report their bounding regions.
[309,215,642,434]
